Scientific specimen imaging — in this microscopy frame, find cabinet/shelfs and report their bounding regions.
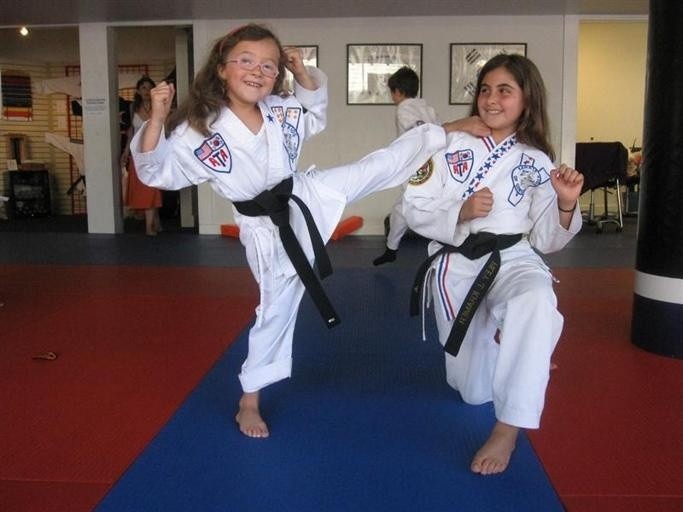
[3,169,52,220]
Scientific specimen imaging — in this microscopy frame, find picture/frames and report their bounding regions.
[347,43,423,106]
[282,45,318,92]
[449,42,527,104]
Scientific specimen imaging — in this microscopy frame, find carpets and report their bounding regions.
[93,267,567,512]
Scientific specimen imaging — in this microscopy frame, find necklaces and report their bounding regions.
[139,102,152,118]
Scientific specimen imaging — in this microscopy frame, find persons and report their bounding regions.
[397,54,584,478]
[129,24,492,438]
[119,76,165,237]
[373,66,439,269]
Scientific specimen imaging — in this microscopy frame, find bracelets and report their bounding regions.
[558,204,578,219]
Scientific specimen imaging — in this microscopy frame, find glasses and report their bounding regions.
[221,53,280,81]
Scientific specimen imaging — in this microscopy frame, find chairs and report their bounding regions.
[575,140,629,232]
